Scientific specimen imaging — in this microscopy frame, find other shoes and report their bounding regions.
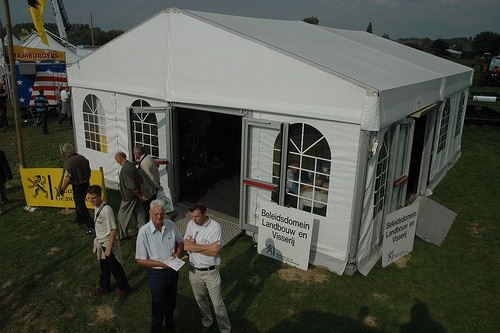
[200,319,215,332]
[116,290,128,298]
[92,287,110,294]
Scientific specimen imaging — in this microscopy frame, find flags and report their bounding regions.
[27,0,50,46]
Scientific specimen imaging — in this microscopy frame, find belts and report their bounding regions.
[194,266,216,271]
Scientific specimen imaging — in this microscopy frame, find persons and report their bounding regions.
[88,185,132,299]
[114,151,146,241]
[59,143,95,234]
[32,88,51,134]
[184,202,232,333]
[135,199,183,333]
[0,150,13,215]
[0,83,9,131]
[56,86,73,126]
[302,175,327,192]
[134,144,163,226]
[55,86,64,120]
[275,160,304,210]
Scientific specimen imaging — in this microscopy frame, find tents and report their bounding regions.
[66,6,474,277]
[1,28,101,124]
